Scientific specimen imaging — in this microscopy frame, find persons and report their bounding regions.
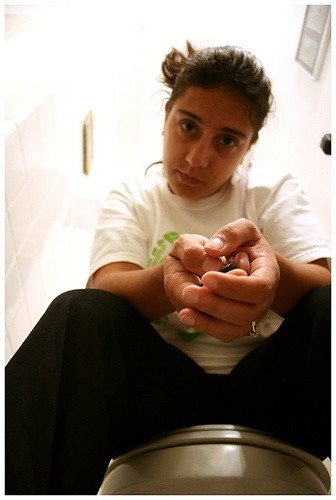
[4,40,331,495]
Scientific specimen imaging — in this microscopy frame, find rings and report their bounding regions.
[249,321,258,338]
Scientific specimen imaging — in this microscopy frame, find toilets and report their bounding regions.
[100,424,331,495]
